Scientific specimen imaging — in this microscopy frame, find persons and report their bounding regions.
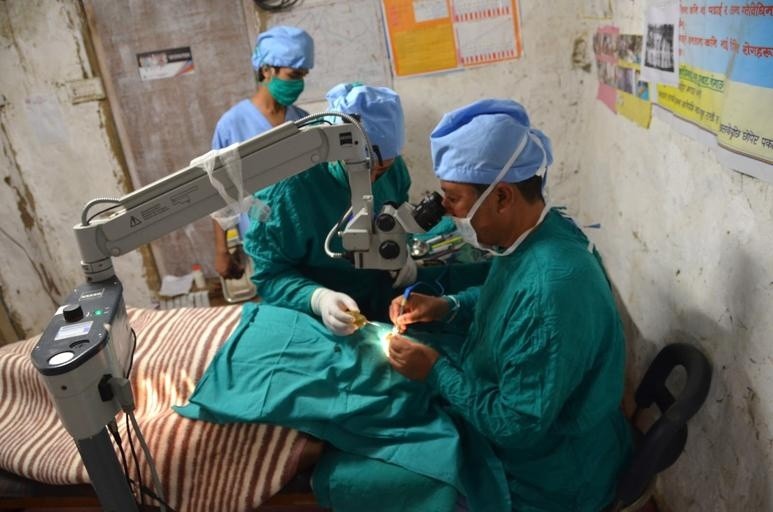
[241,81,496,338]
[387,97,637,511]
[209,25,316,242]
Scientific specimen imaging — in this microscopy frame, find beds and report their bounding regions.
[0,306,462,512]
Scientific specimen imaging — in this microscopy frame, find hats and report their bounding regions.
[251,26,314,71]
[429,98,552,184]
[326,83,404,162]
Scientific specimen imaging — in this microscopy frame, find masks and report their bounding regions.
[260,64,304,107]
[452,182,503,257]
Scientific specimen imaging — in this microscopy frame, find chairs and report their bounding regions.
[601,342,711,510]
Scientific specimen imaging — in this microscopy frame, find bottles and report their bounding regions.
[192,265,206,289]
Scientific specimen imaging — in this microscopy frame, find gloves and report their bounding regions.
[311,287,360,337]
